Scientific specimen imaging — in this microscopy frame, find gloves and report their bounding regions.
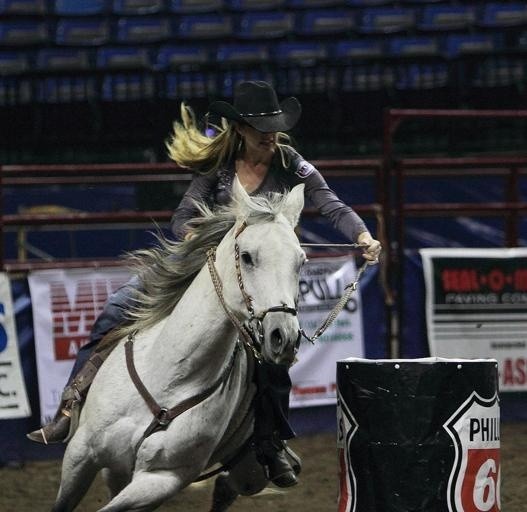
[357,232,382,265]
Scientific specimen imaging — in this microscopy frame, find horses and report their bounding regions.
[50,173,309,512]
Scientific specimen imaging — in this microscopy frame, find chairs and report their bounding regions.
[0,1,527,103]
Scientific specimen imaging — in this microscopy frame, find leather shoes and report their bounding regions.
[269,453,298,488]
[26,416,71,445]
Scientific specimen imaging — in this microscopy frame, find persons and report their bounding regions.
[27,80,382,488]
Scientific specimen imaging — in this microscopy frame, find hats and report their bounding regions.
[209,81,302,134]
